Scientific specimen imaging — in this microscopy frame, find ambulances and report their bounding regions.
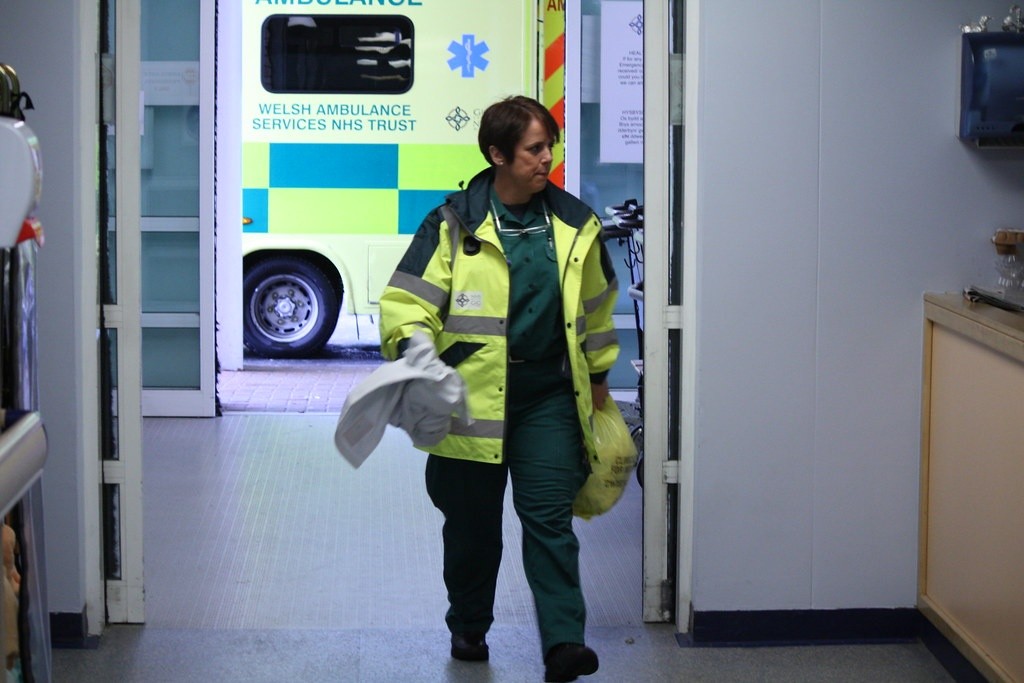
[236,0,564,361]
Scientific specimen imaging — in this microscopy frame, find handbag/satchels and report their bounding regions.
[571,395,638,518]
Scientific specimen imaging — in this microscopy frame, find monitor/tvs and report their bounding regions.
[959,33,1024,140]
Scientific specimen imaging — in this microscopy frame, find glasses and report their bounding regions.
[489,192,551,236]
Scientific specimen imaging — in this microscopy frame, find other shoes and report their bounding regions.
[451,631,489,662]
[544,643,599,683]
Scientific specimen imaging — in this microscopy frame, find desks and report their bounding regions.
[924,292,1024,683]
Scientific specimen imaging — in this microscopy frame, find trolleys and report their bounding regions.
[599,196,645,490]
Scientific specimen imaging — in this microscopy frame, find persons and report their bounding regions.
[377,97,618,683]
